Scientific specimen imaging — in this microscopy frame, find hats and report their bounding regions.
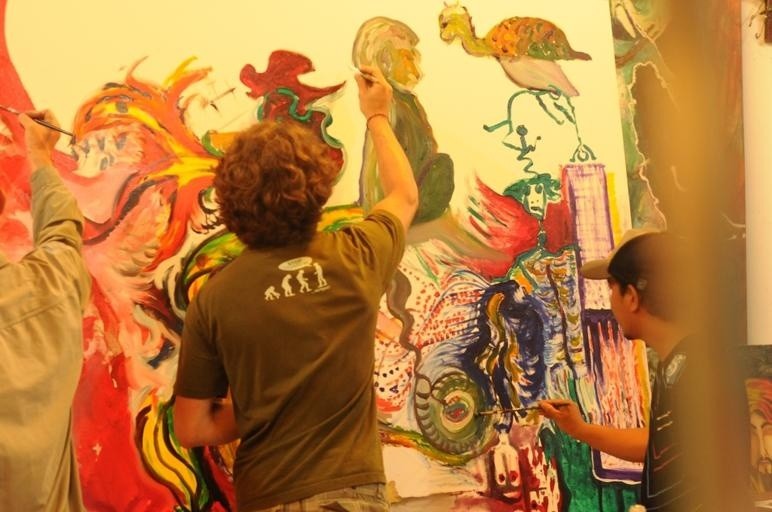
[579,228,698,285]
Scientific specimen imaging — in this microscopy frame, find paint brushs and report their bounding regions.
[473,403,571,417]
[347,64,382,86]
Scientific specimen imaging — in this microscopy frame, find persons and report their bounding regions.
[534,230,754,512]
[172,63,421,511]
[0,106,95,511]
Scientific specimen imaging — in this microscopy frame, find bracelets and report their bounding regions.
[366,112,389,130]
